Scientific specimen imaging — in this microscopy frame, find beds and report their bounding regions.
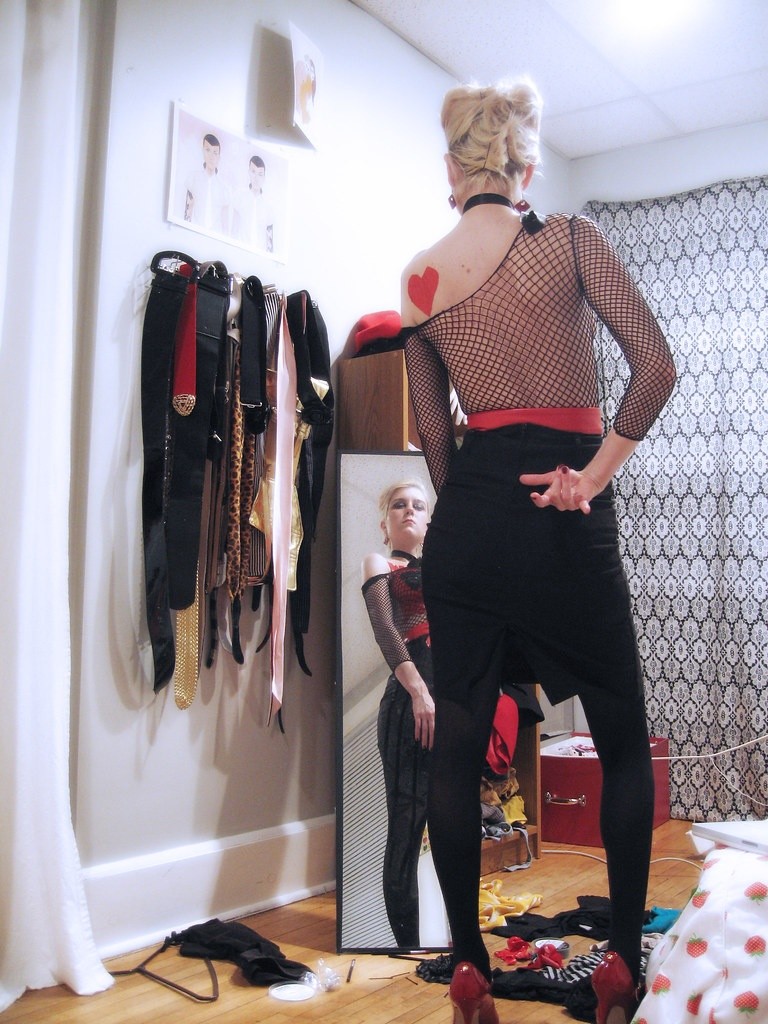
[627,838,768,1024]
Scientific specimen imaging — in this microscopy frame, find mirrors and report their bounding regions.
[338,449,453,955]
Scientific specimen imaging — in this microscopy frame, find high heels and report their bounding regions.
[590,953,649,1024]
[447,961,499,1024]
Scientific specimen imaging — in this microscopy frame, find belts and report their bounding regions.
[139,250,334,733]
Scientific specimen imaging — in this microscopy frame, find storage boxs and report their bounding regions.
[541,731,670,849]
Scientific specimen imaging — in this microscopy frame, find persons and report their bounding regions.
[403,72,675,1024]
[362,484,435,944]
[186,134,272,248]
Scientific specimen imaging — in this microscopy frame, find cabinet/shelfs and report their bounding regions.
[338,348,542,880]
